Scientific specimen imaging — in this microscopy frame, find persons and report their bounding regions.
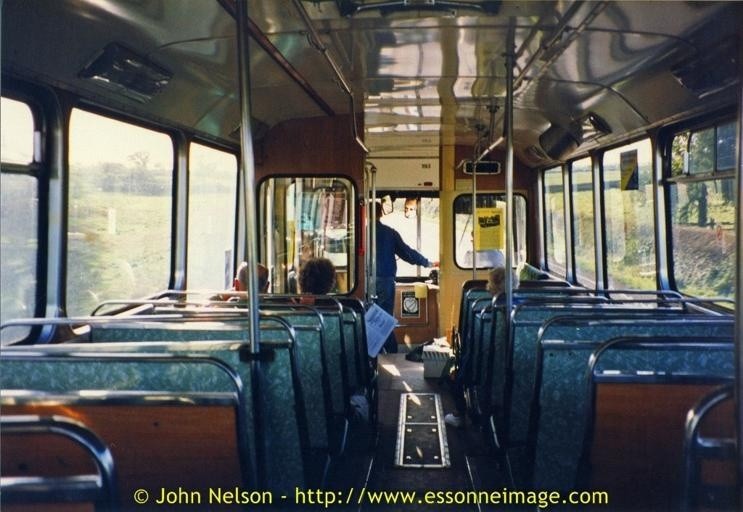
[461,230,505,268]
[216,263,270,303]
[405,200,415,217]
[317,200,440,353]
[291,257,335,307]
[443,267,525,429]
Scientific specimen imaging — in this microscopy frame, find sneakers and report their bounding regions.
[446,413,464,428]
[350,395,368,421]
